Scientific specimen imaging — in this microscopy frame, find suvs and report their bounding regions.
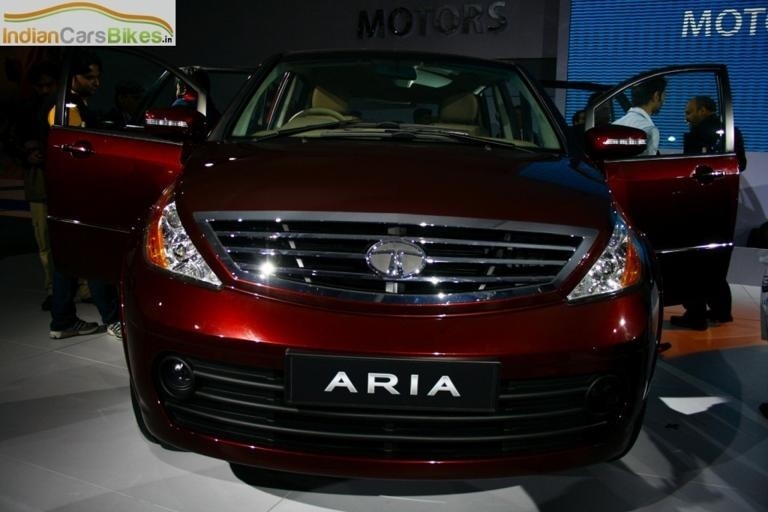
[44,44,740,481]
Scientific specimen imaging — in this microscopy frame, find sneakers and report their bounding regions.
[50,317,100,339]
[108,322,121,338]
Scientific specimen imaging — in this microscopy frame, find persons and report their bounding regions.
[104,81,149,132]
[157,70,222,143]
[670,95,746,330]
[608,75,667,160]
[572,110,586,126]
[567,92,612,147]
[515,105,540,147]
[41,53,125,338]
[11,57,97,309]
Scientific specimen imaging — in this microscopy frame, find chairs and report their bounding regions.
[289,78,481,135]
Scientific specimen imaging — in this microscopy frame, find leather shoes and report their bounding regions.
[670,314,708,331]
[709,311,734,322]
